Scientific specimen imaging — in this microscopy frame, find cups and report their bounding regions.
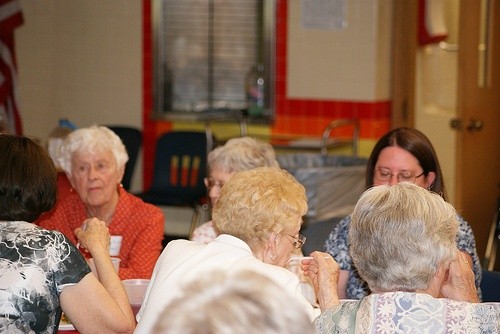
[89,257,120,282]
[108,236,122,256]
[121,279,151,324]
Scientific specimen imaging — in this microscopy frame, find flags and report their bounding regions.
[0,0,24,136]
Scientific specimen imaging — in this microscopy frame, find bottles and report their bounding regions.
[248,62,265,115]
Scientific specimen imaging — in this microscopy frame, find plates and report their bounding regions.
[57,325,74,331]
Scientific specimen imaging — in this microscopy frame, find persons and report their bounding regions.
[33,125,164,279]
[326,127,482,302]
[0,133,137,334]
[189,136,317,305]
[312,182,500,334]
[134,166,341,334]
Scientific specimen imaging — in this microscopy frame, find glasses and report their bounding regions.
[74,160,111,177]
[374,170,425,183]
[282,233,307,249]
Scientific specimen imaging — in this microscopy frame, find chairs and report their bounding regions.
[94,124,143,192]
[137,123,216,241]
